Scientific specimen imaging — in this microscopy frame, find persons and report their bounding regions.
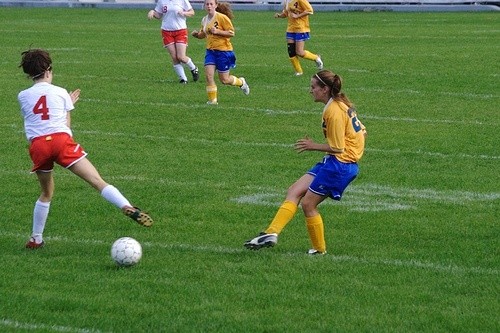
[244,68,367,256]
[17,49,154,250]
[147,0,200,85]
[274,0,323,76]
[191,0,249,105]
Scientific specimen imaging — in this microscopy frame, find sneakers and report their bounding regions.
[123,205,153,227]
[306,249,328,255]
[191,67,200,82]
[240,77,250,96]
[179,79,188,84]
[26,233,44,248]
[315,57,324,69]
[243,232,277,250]
[296,73,301,75]
[207,101,217,105]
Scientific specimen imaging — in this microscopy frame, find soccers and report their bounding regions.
[110,236,143,267]
